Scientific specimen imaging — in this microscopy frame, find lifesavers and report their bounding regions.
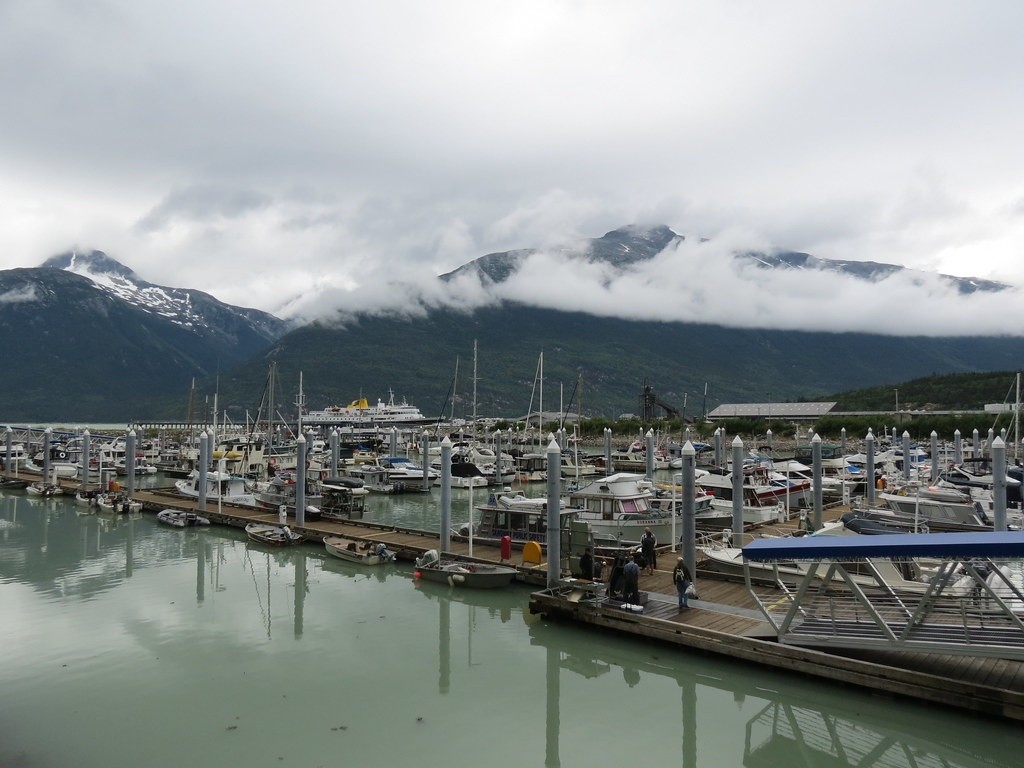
[270,458,276,465]
[306,460,310,469]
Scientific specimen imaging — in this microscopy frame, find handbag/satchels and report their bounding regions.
[624,563,636,586]
[685,582,696,596]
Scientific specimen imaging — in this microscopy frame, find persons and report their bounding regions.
[673,557,693,608]
[623,556,640,604]
[640,527,657,577]
[579,548,596,598]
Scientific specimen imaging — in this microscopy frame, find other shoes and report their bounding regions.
[679,604,689,608]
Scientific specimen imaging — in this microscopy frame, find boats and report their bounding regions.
[450,447,933,558]
[157,508,211,530]
[323,534,398,566]
[407,549,520,591]
[26,481,63,497]
[695,528,976,611]
[98,491,143,514]
[72,481,108,508]
[297,388,446,427]
[0,339,697,512]
[785,461,1023,598]
[1,476,26,490]
[245,522,304,548]
[254,473,342,518]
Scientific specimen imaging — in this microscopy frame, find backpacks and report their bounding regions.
[675,568,684,582]
